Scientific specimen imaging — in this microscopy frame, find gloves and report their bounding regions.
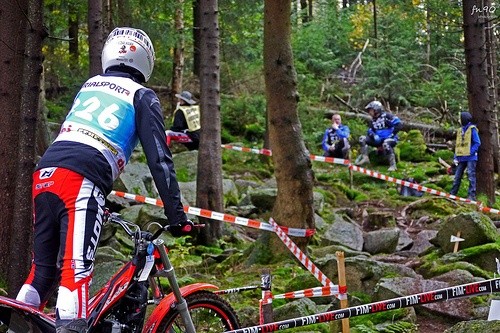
[388,134,393,138]
[373,135,380,143]
[169,219,195,238]
[454,159,459,166]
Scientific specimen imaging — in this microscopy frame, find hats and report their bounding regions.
[175,91,196,105]
[461,112,472,126]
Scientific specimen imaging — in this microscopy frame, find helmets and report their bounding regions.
[365,101,384,111]
[102,27,154,82]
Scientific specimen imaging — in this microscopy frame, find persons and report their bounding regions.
[7,27,188,333]
[447,111,481,201]
[171,90,201,151]
[322,114,350,166]
[356,101,405,172]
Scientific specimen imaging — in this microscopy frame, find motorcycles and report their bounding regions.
[0,205,243,333]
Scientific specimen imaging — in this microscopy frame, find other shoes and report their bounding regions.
[467,196,476,201]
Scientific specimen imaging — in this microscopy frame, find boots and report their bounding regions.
[357,147,370,165]
[387,153,397,171]
